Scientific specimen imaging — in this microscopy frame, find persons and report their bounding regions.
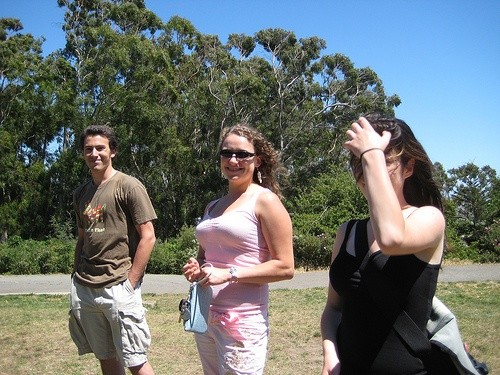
[182,126,295,375]
[71,125,158,375]
[320,115,445,375]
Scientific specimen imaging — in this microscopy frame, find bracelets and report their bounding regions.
[229,266,239,284]
[359,148,384,162]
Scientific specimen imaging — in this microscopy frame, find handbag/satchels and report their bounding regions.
[421,294,488,375]
[180,263,212,333]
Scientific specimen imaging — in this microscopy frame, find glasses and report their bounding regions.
[220,149,256,160]
